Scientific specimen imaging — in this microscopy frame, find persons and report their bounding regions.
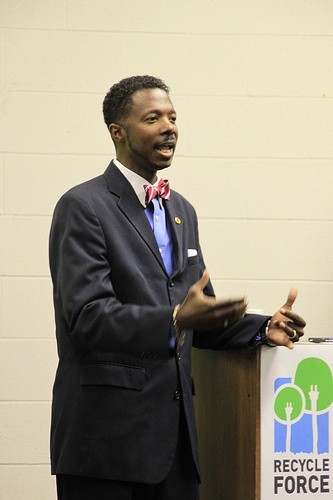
[47,74,306,500]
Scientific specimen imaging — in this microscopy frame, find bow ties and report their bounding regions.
[142,178,171,206]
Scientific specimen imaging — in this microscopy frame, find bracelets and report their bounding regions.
[172,303,186,347]
[266,319,276,347]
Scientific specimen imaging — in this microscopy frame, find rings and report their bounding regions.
[288,329,296,339]
